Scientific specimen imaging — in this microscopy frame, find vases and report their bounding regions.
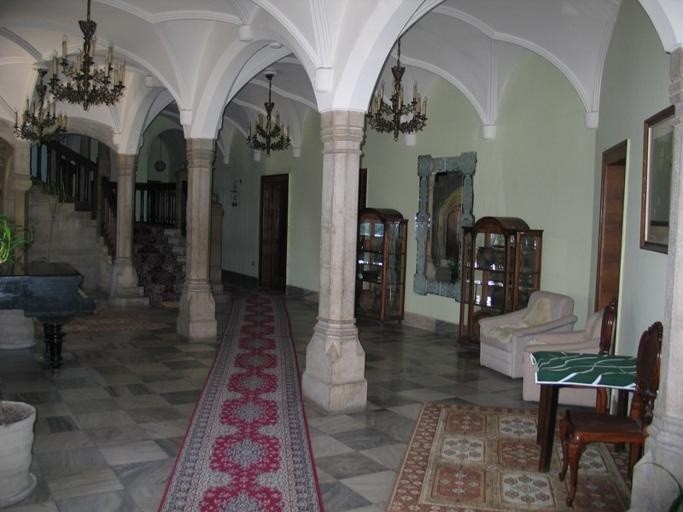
[0,400,39,507]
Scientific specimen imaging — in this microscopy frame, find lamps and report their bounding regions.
[367,37,428,142]
[12,0,123,151]
[241,72,292,158]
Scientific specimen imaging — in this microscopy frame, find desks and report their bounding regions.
[532,349,637,474]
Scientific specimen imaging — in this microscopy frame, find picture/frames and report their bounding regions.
[640,103,675,256]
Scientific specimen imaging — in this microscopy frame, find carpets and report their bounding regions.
[385,395,632,511]
[156,289,325,511]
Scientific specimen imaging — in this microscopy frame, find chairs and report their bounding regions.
[557,321,664,505]
[477,289,618,415]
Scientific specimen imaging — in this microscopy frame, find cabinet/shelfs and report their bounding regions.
[456,215,542,347]
[352,206,407,325]
